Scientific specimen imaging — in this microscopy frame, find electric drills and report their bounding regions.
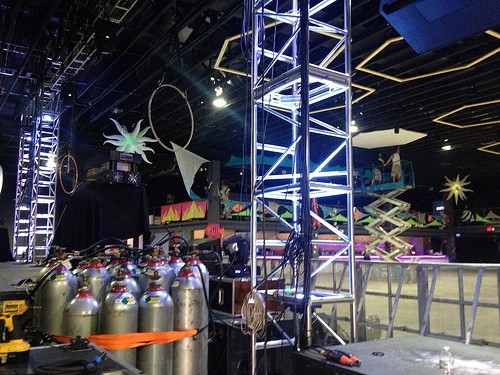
[0,300,42,342]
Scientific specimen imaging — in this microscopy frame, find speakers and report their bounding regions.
[179,237,222,260]
[446,224,500,263]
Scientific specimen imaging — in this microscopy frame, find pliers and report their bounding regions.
[309,344,363,367]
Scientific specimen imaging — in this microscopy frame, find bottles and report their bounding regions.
[366,315,380,339]
[438,346,455,375]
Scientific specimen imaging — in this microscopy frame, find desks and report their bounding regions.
[295,334,500,375]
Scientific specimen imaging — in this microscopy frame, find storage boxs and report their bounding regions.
[1,338,146,375]
[210,275,287,318]
[209,317,295,375]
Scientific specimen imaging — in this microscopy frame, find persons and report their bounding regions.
[428,215,441,255]
[371,152,384,185]
[383,146,400,182]
